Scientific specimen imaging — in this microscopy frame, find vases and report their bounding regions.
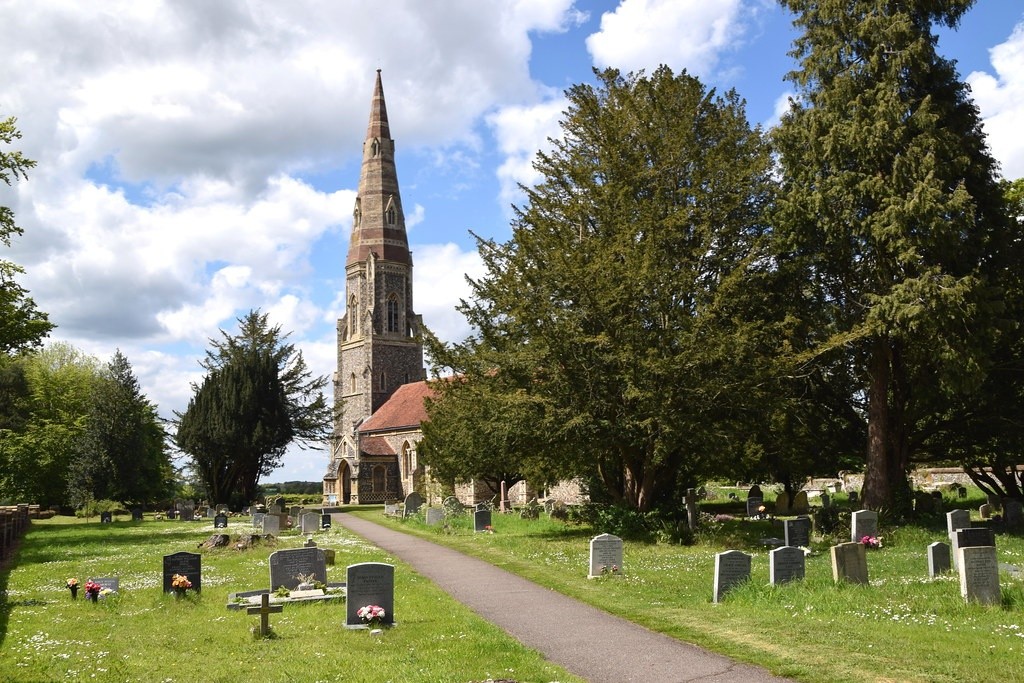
[176,588,186,596]
[71,589,77,598]
[91,594,98,603]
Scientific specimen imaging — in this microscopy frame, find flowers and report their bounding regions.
[65,578,81,589]
[357,604,385,622]
[171,574,192,589]
[85,579,101,600]
